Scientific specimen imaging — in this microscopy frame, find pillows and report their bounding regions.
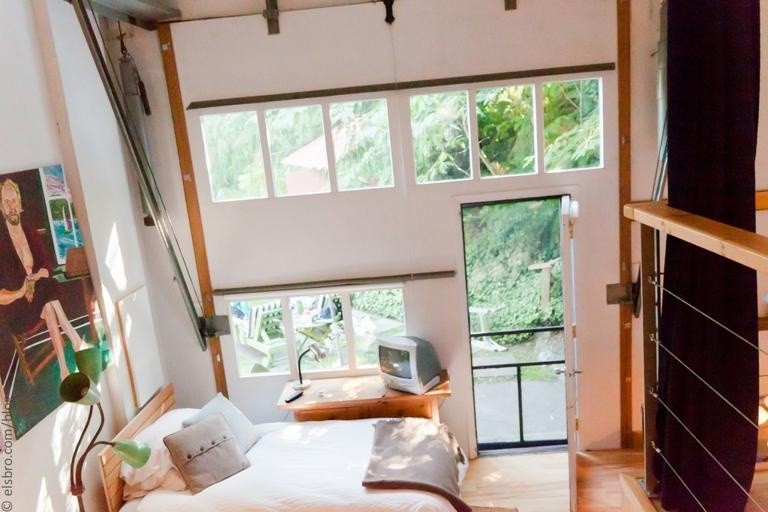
[162,412,250,495]
[183,393,265,457]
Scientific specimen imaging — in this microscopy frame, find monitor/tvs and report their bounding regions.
[375,335,442,397]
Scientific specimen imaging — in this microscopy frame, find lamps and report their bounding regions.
[292,342,326,389]
[58,347,151,512]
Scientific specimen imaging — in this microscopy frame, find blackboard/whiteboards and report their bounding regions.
[117,285,164,409]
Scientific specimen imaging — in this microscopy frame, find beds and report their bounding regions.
[97,384,469,512]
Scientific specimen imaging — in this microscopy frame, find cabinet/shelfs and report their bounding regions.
[278,370,452,419]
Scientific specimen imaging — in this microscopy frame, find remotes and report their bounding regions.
[285,390,304,403]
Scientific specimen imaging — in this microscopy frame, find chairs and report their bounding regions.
[233,302,288,359]
